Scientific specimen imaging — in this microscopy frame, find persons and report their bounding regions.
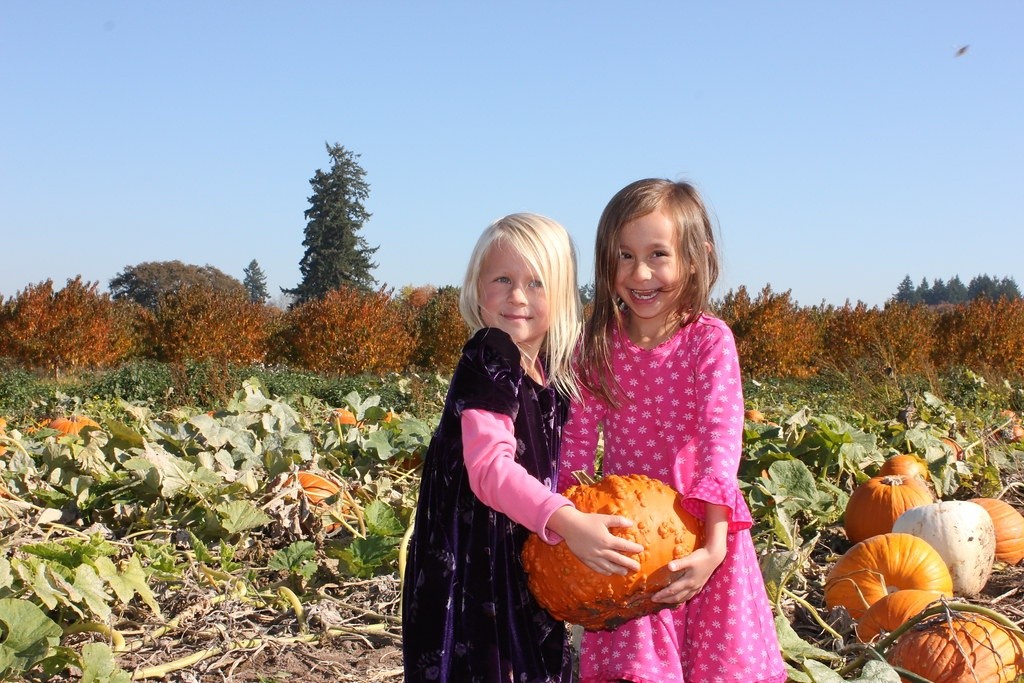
[402,212,643,683]
[555,178,787,683]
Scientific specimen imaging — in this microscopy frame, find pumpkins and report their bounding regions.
[522,411,1024,683]
[0,407,423,538]
